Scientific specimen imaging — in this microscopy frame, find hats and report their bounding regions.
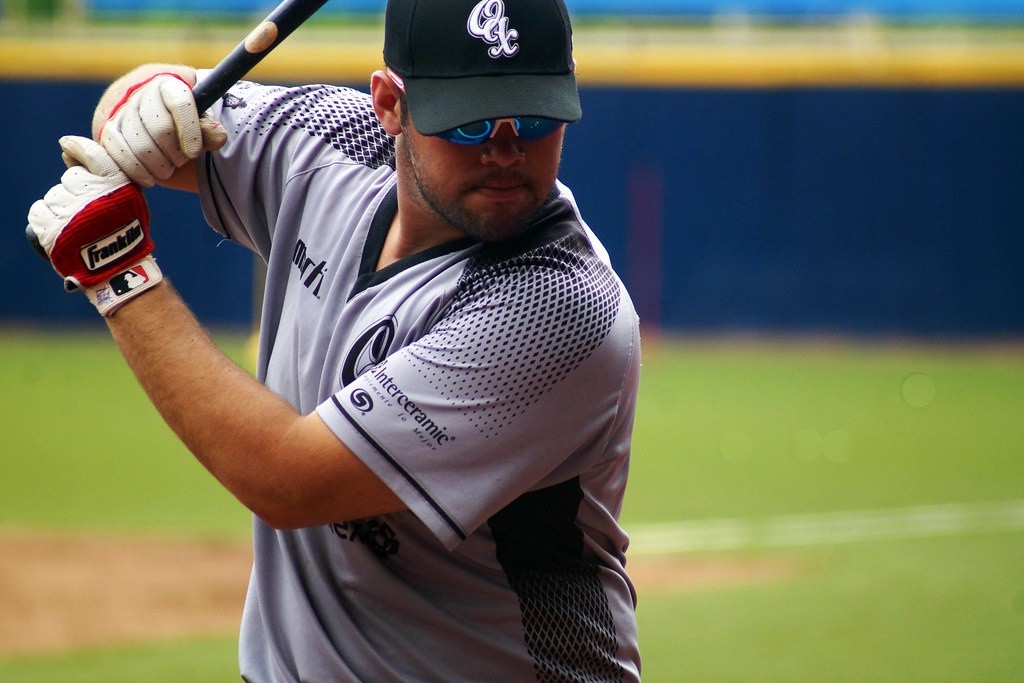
[383,0,582,135]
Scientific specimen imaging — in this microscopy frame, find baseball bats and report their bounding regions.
[20,0,333,266]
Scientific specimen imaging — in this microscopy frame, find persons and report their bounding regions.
[28,0,647,683]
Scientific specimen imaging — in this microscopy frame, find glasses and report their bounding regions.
[386,68,566,146]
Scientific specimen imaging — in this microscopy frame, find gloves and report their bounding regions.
[91,63,228,188]
[28,135,162,317]
